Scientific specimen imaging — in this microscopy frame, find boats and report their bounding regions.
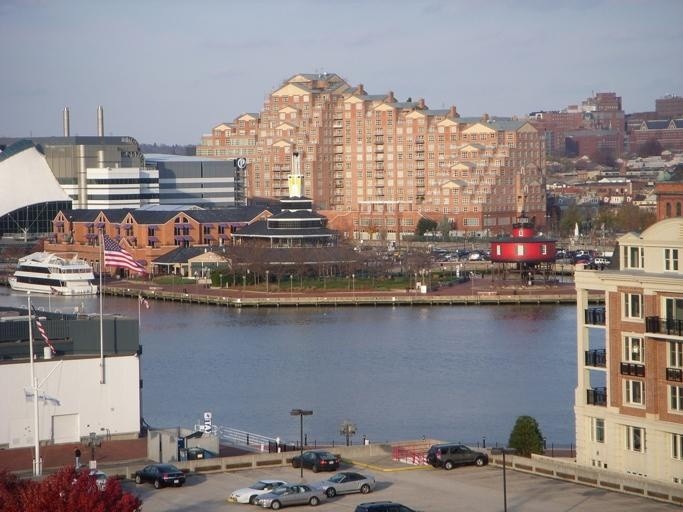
[8,252,97,295]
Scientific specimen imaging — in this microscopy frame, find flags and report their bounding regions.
[32,305,56,357]
[139,296,149,309]
[104,234,146,274]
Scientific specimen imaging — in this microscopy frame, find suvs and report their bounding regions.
[427,444,488,470]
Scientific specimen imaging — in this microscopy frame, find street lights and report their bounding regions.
[340,424,357,446]
[289,275,294,297]
[491,446,506,512]
[290,408,313,478]
[346,274,355,292]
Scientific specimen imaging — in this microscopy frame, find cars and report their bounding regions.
[135,463,185,489]
[431,247,491,262]
[228,470,376,510]
[88,472,108,493]
[292,450,339,473]
[354,501,416,512]
[555,250,614,264]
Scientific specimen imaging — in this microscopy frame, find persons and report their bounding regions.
[72,446,82,469]
